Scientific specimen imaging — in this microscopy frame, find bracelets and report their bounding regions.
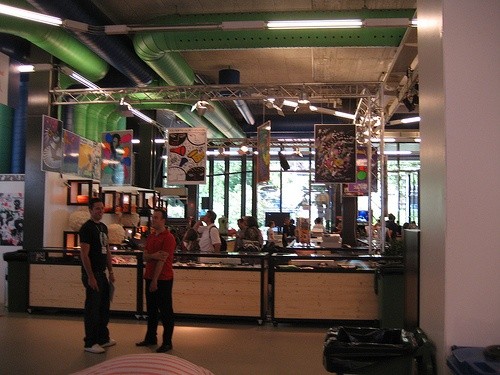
[109,271,113,274]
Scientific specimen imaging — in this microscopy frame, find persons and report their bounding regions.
[233,216,263,252]
[193,210,221,252]
[78,198,117,353]
[135,208,176,353]
[312,217,324,233]
[177,229,200,263]
[331,220,343,239]
[372,214,419,244]
[282,218,297,248]
[266,221,275,243]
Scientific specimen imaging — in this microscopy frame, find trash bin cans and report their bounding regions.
[323,325,417,375]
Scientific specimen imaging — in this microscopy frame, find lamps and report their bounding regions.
[278,151,290,172]
[402,98,414,112]
[413,95,419,104]
[61,66,166,131]
[265,18,365,29]
[191,100,214,117]
[219,67,240,97]
[263,98,355,119]
[0,4,62,27]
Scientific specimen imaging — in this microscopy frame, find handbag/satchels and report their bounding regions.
[209,226,227,251]
[241,239,260,252]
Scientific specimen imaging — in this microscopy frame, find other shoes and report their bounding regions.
[156,345,173,353]
[100,339,116,347]
[136,340,157,346]
[84,344,105,353]
